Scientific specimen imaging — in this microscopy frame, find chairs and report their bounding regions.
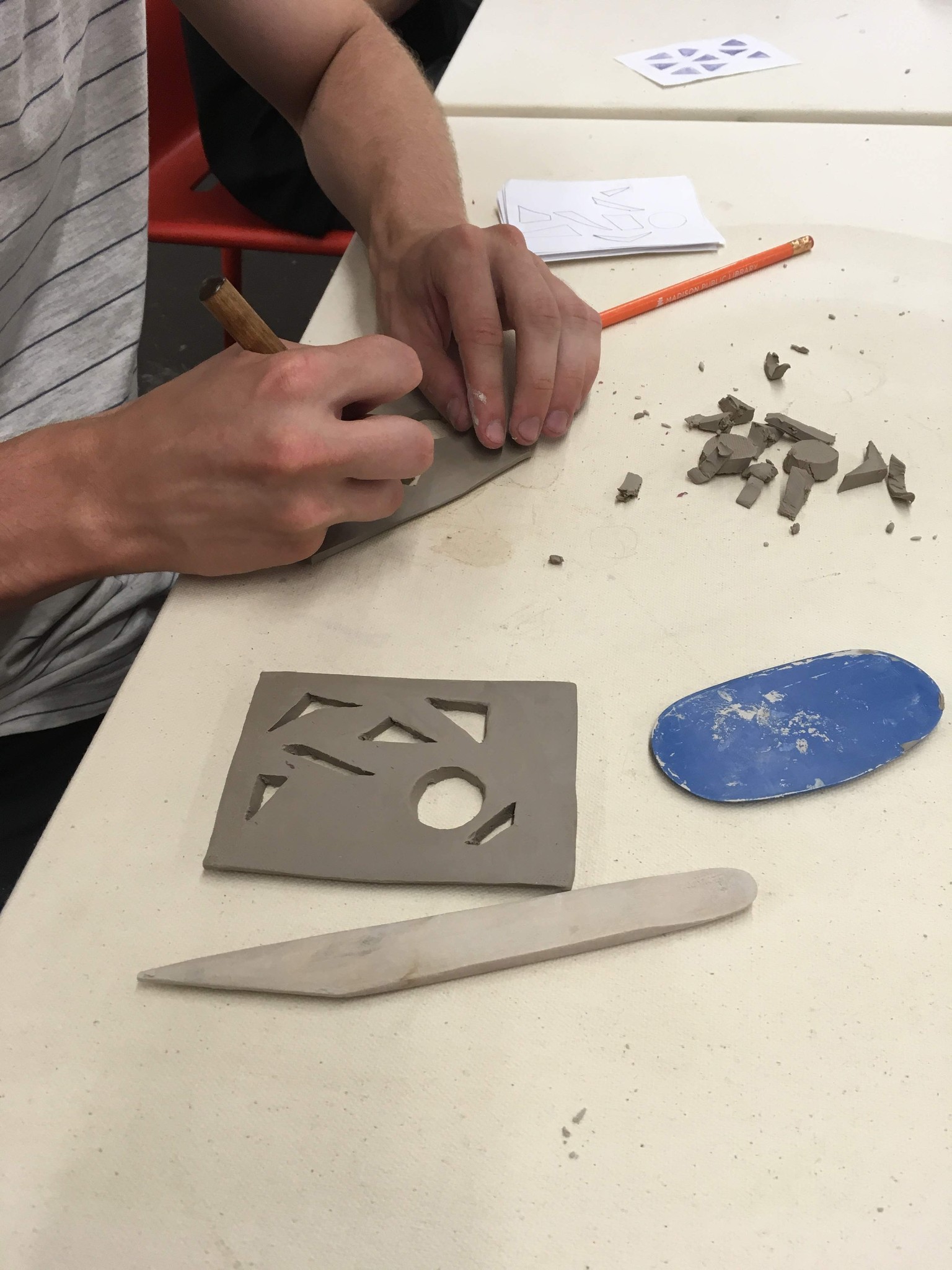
[146,1,354,348]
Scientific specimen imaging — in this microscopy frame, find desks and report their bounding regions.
[0,117,952,1270]
[430,0,952,126]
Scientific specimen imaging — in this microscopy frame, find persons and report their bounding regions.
[0,0,602,912]
[175,0,482,242]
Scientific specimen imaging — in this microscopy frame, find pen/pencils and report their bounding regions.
[598,236,814,326]
